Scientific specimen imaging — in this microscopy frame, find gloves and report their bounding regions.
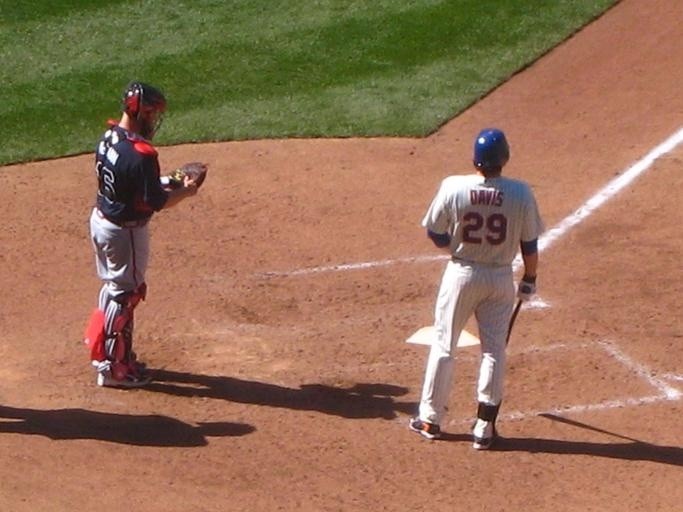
[516,273,536,304]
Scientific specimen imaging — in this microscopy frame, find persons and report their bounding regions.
[84,81,199,389]
[410,127,539,448]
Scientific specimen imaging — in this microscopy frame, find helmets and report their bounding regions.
[472,128,509,170]
[120,79,166,141]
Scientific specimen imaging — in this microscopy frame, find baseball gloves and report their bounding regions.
[165,161,207,192]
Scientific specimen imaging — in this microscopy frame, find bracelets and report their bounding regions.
[524,276,537,283]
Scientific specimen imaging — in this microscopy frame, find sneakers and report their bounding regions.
[98,366,152,388]
[469,420,497,452]
[408,418,443,439]
